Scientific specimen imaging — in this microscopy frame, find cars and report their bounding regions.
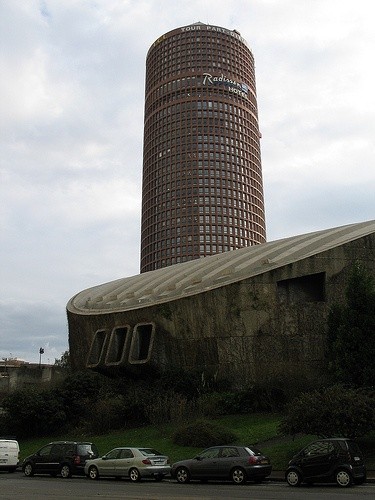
[170,445,273,485]
[84,446,171,482]
[285,437,367,488]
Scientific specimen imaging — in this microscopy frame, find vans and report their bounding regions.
[22,440,99,479]
[0,440,20,471]
